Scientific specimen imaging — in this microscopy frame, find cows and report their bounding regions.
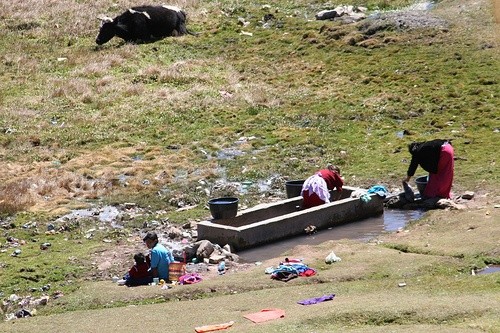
[96,5,199,45]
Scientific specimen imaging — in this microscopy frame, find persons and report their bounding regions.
[142,233,174,281]
[129,253,153,283]
[301,165,343,209]
[405,138,454,200]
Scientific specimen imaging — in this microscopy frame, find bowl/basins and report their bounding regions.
[208,197,239,218]
[286,180,306,198]
[415,175,428,193]
